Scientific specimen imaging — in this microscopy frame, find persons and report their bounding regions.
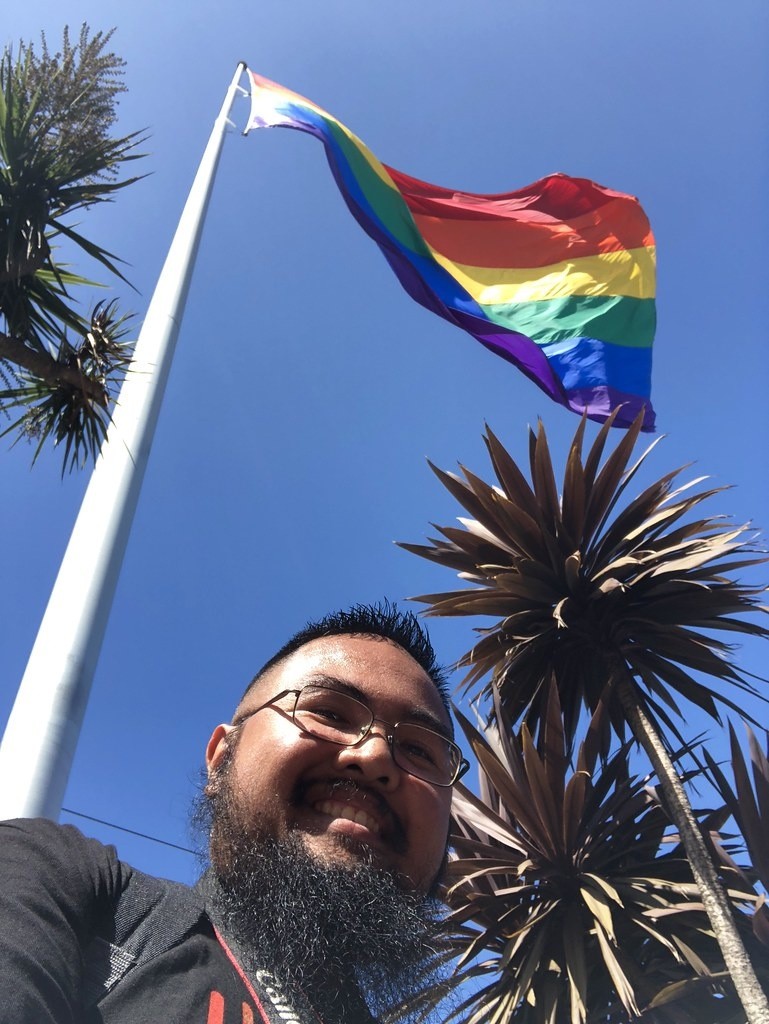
[0,597,471,1024]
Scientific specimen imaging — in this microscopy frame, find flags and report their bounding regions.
[245,69,660,436]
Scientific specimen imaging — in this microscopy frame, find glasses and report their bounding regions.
[234,684,470,788]
